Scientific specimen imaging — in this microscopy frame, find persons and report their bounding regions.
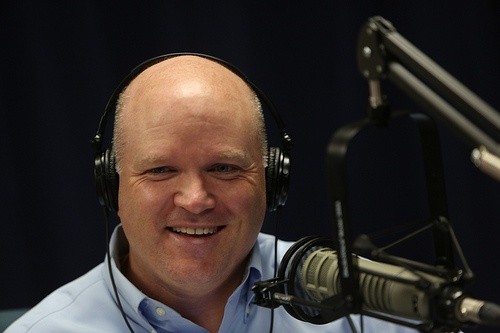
[1,55,422,333]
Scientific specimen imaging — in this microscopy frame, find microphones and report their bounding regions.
[296,246,500,329]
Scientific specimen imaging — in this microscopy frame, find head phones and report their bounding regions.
[92,53,294,214]
